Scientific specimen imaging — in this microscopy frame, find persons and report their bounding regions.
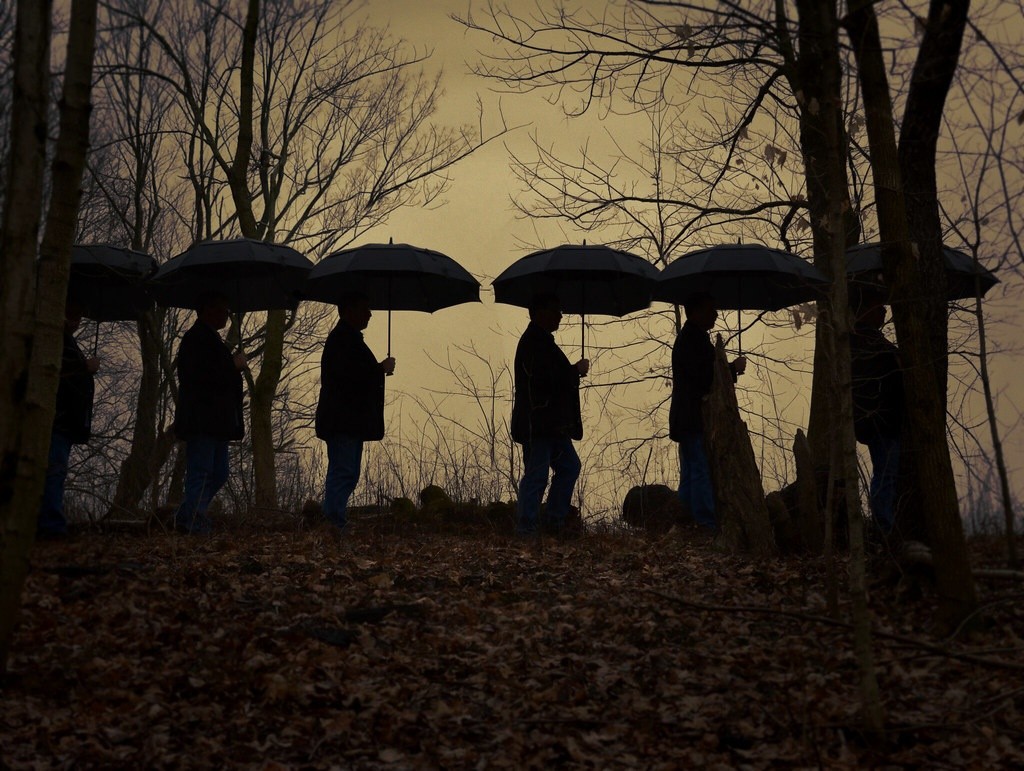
[844,302,909,534]
[165,289,249,535]
[314,291,393,529]
[32,292,101,534]
[669,283,747,529]
[511,292,591,539]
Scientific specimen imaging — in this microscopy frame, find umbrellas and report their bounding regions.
[840,232,1001,301]
[293,237,483,379]
[153,235,316,360]
[491,238,661,377]
[28,244,161,360]
[658,237,829,377]
